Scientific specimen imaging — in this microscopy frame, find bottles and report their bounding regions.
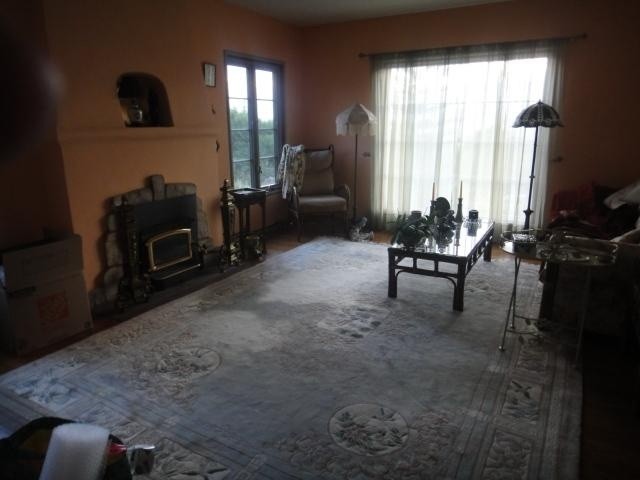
[126,102,144,126]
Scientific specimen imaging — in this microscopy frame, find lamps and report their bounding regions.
[334,102,377,234]
[506,101,563,255]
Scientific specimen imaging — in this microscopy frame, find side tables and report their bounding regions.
[497,237,618,364]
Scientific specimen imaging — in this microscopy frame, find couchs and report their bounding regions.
[536,188,640,361]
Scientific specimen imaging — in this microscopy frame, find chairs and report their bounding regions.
[284,144,350,243]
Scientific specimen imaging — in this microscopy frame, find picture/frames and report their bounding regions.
[203,63,216,87]
